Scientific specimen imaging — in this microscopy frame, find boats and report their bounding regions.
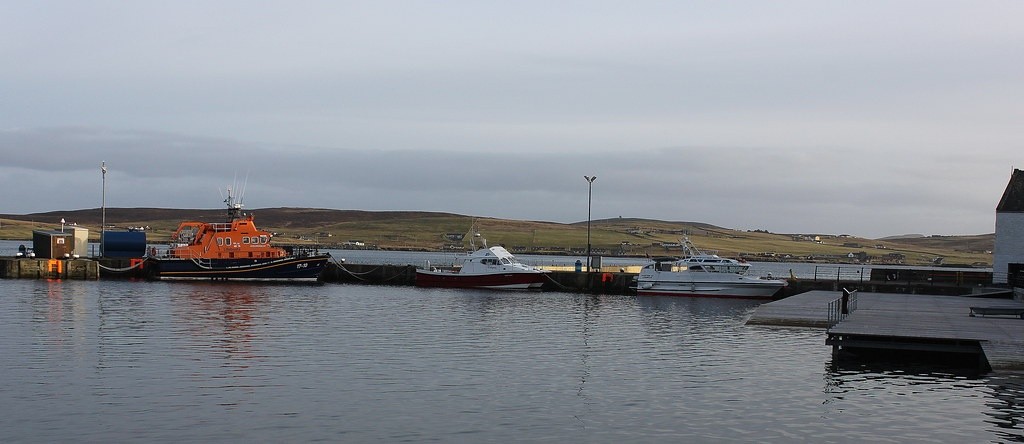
[151,189,332,284]
[627,234,788,298]
[416,231,547,291]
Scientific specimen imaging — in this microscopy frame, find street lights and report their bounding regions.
[101,161,107,259]
[584,175,597,272]
